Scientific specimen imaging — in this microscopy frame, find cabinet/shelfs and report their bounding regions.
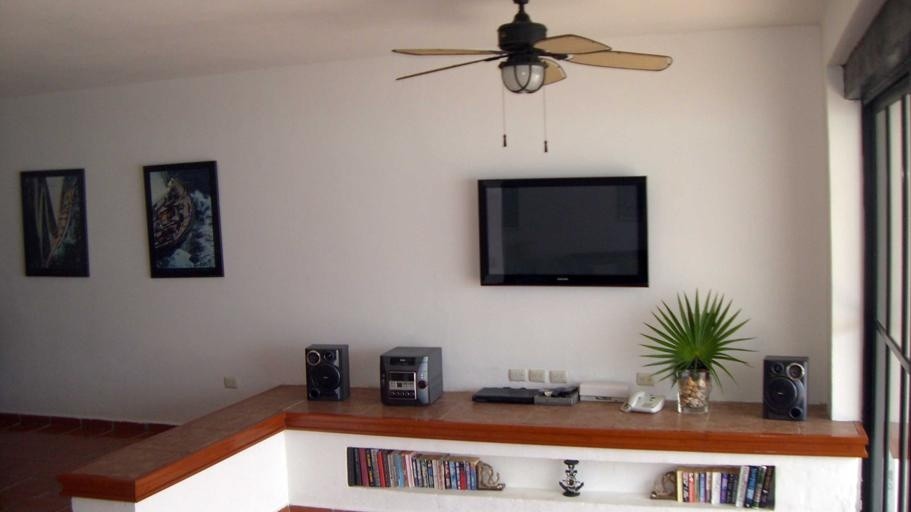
[58,382,871,511]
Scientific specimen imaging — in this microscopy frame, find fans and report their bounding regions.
[390,0,674,86]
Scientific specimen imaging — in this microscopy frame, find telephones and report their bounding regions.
[627,390,666,414]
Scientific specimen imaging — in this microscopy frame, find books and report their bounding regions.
[348,447,480,490]
[676,466,774,509]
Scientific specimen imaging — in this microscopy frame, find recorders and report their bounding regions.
[380,346,442,407]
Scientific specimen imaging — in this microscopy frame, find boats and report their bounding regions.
[149,171,197,254]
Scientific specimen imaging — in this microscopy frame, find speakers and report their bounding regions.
[305,344,350,401]
[762,355,808,421]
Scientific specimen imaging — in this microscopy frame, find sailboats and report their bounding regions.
[30,173,74,269]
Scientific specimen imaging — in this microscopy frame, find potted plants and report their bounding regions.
[637,289,758,414]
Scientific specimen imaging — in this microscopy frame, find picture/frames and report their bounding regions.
[18,168,90,278]
[142,160,224,278]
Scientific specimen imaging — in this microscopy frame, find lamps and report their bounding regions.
[498,54,549,154]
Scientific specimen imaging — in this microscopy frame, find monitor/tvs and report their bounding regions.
[478,176,649,288]
